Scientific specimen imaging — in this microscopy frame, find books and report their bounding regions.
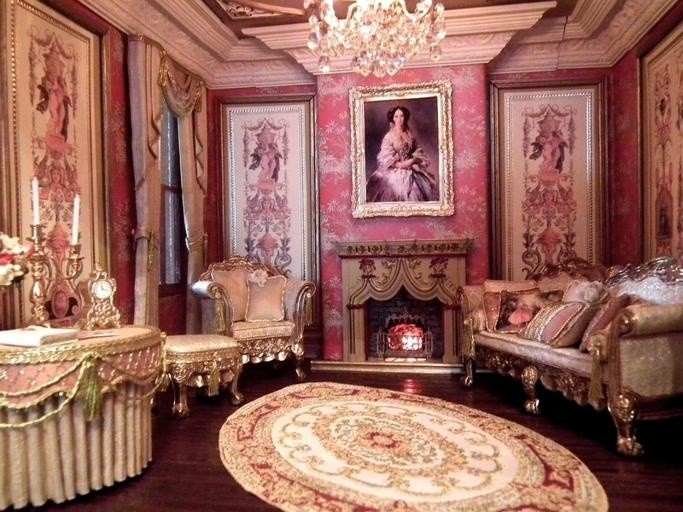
[0,326,78,347]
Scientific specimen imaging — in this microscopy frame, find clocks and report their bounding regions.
[84,263,119,329]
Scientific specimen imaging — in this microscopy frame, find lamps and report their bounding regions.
[302,1,447,77]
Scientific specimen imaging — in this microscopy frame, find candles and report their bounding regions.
[69,194,81,247]
[29,176,42,227]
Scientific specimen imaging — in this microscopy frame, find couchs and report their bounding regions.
[191,257,316,382]
[459,258,683,459]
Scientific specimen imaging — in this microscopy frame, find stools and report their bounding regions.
[152,333,243,419]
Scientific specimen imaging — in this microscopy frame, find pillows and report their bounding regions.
[519,300,589,348]
[558,278,600,306]
[578,285,628,354]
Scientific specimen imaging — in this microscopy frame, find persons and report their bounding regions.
[366,106,439,202]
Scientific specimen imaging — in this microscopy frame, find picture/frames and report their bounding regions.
[1,1,111,331]
[220,96,314,328]
[349,80,455,219]
[491,76,611,283]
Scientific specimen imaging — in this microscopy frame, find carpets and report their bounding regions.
[220,381,610,512]
